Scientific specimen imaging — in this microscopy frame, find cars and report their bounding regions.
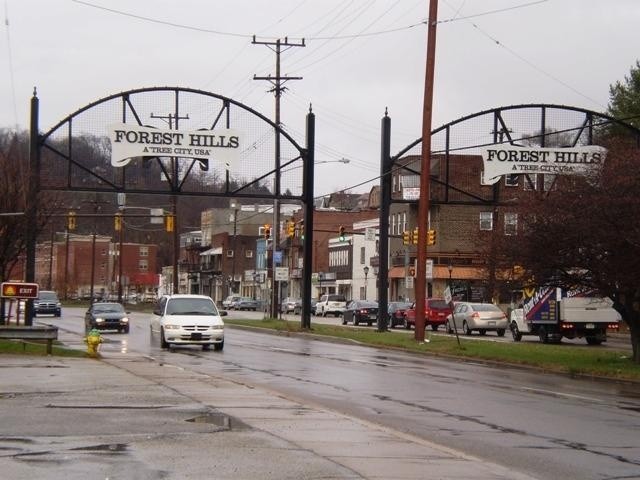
[149,294,228,351]
[70,284,158,302]
[17,290,61,317]
[85,302,131,333]
[221,294,508,337]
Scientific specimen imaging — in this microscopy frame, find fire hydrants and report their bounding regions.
[83,326,104,357]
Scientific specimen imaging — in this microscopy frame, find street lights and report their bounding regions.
[109,249,119,281]
[363,266,369,299]
[318,271,323,294]
[274,158,350,319]
[447,262,453,294]
[119,206,179,294]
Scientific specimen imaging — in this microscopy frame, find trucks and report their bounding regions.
[510,267,622,344]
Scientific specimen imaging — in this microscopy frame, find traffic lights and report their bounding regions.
[403,228,436,245]
[338,225,345,243]
[288,222,304,239]
[265,224,270,239]
